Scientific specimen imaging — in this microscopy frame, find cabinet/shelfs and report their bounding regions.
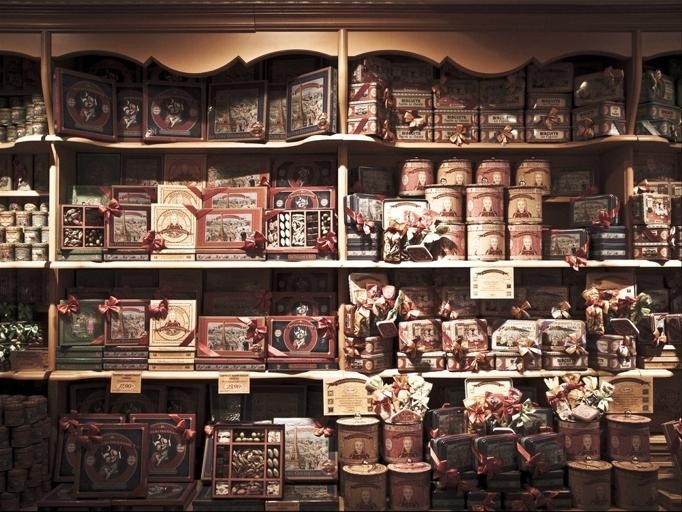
[639,2,681,511]
[0,0,51,511]
[343,0,639,512]
[51,1,343,511]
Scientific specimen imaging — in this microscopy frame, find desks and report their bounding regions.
[36,480,201,512]
